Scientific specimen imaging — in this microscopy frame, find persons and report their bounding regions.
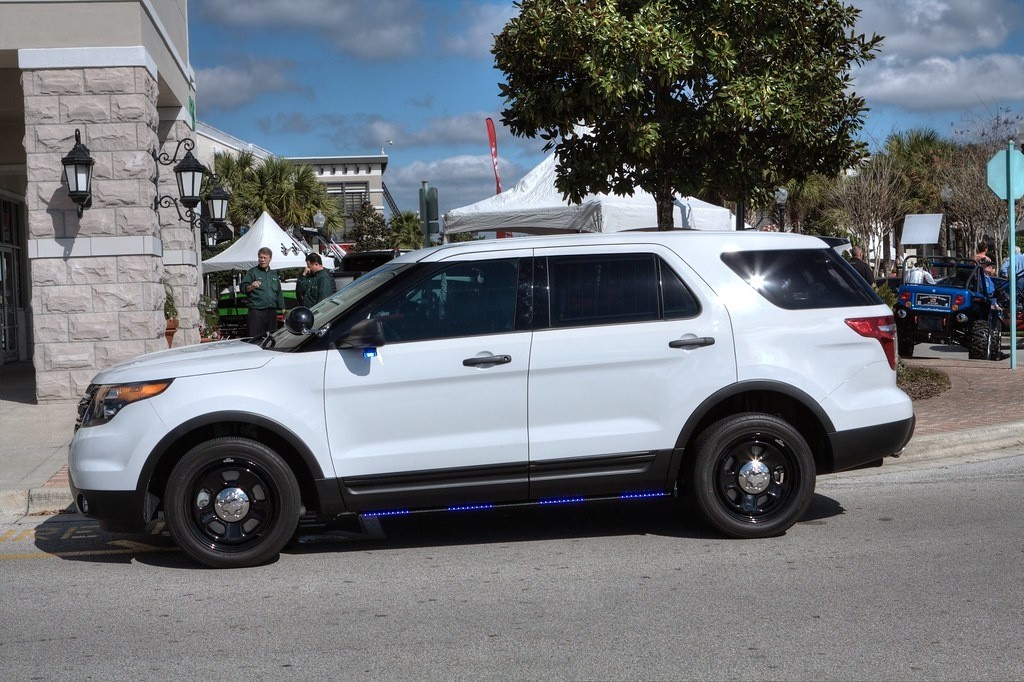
[1000,246,1024,305]
[240,247,285,338]
[988,264,997,278]
[978,257,1001,323]
[971,241,992,267]
[903,262,937,286]
[296,252,338,309]
[845,245,876,288]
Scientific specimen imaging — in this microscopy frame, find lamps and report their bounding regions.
[195,173,232,239]
[153,138,209,231]
[62,127,96,218]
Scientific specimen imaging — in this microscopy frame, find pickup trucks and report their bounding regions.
[327,247,418,302]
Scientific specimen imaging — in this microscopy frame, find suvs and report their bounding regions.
[890,254,1005,361]
[66,226,919,568]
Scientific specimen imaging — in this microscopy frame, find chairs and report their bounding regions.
[216,327,248,340]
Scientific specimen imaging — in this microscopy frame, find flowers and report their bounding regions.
[163,292,176,321]
[198,294,218,339]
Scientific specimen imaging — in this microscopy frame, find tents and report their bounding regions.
[201,209,338,319]
[436,105,757,323]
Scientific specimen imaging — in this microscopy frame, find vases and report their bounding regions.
[165,320,179,347]
[200,337,214,345]
